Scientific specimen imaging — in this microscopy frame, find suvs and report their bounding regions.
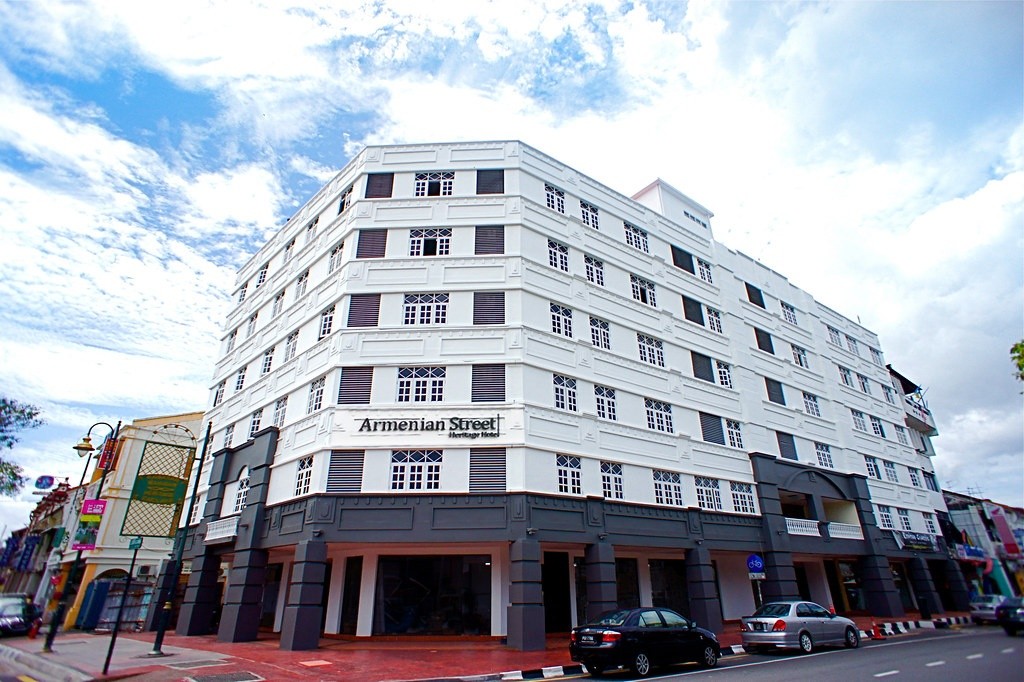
[969,595,1024,636]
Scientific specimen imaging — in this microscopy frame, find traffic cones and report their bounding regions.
[871,616,886,640]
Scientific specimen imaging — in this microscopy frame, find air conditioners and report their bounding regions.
[137,565,156,577]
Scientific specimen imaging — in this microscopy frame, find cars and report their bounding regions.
[0,593,32,637]
[569,607,722,678]
[741,601,861,655]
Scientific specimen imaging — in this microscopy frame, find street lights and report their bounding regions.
[43,421,123,653]
